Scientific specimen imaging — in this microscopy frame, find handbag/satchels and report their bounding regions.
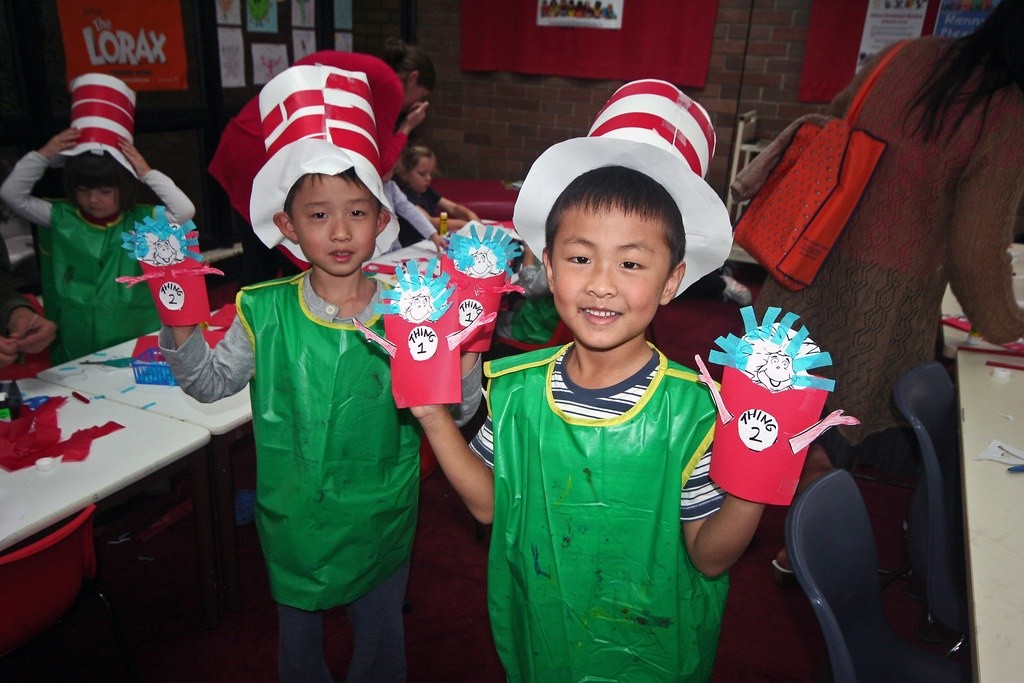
[731,36,917,293]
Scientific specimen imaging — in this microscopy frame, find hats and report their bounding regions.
[249,63,401,263]
[512,81,734,302]
[57,73,141,180]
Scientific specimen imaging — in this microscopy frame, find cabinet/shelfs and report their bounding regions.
[726,111,768,263]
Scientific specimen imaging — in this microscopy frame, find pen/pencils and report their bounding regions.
[1008,464,1024,471]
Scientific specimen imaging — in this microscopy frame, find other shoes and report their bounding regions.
[770,550,802,587]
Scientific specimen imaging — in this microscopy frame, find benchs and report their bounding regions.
[424,178,524,220]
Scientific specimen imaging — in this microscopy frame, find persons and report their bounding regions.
[211,47,437,296]
[548,0,559,17]
[583,0,595,17]
[575,0,585,17]
[749,0,1024,587]
[567,0,575,17]
[600,3,617,19]
[407,78,836,683]
[380,170,448,255]
[540,0,549,17]
[391,143,483,249]
[137,53,522,683]
[0,71,195,368]
[592,0,602,18]
[559,0,568,17]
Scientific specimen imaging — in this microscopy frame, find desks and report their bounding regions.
[0,304,256,629]
[941,280,1024,683]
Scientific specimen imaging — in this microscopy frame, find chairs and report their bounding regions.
[491,320,575,351]
[0,503,112,658]
[783,362,976,683]
